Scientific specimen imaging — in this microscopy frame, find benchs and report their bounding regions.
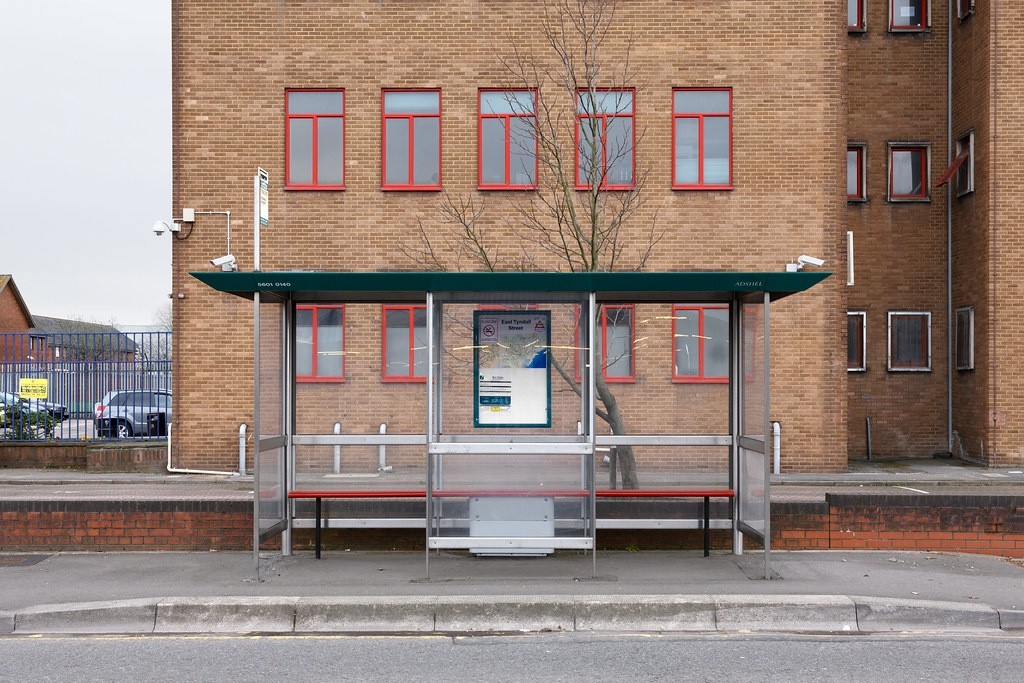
[288,489,735,558]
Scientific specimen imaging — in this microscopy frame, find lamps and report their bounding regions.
[153,221,180,237]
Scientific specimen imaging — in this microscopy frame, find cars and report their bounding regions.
[0,392,49,424]
[9,392,70,423]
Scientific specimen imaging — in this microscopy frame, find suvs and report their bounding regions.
[92,388,172,437]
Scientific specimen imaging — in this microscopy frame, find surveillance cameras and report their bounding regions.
[210,254,236,267]
[799,255,825,266]
[153,222,165,236]
